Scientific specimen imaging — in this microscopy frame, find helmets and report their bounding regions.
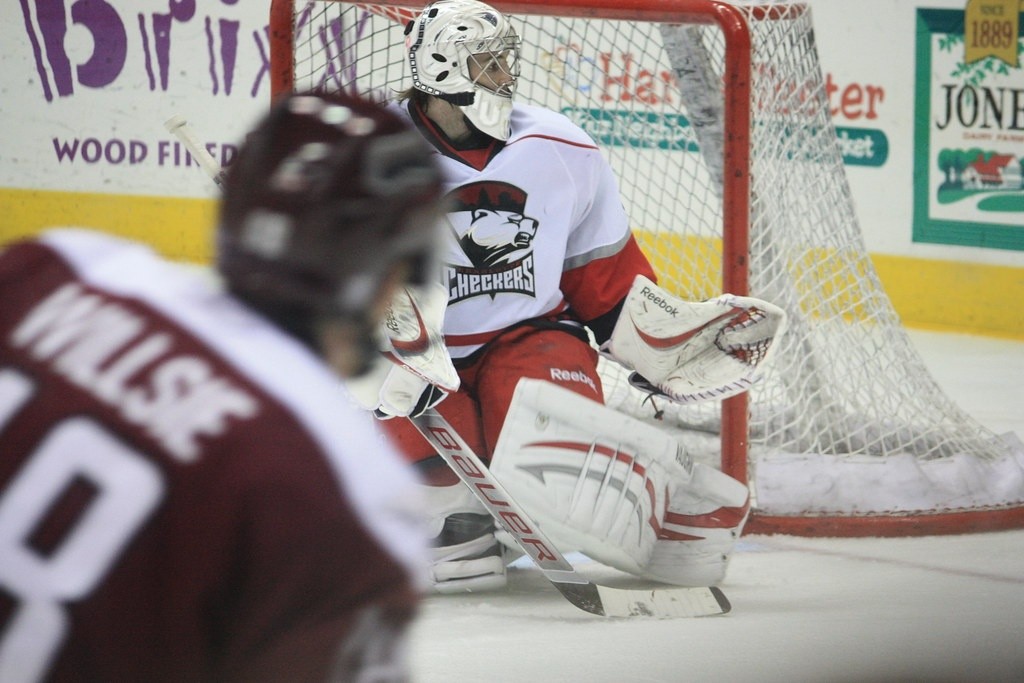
[404,0,522,142]
[217,92,448,311]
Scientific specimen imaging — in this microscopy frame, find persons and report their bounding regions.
[345,0,787,592]
[1,90,449,683]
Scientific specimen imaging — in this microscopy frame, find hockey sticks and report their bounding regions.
[160,111,735,622]
